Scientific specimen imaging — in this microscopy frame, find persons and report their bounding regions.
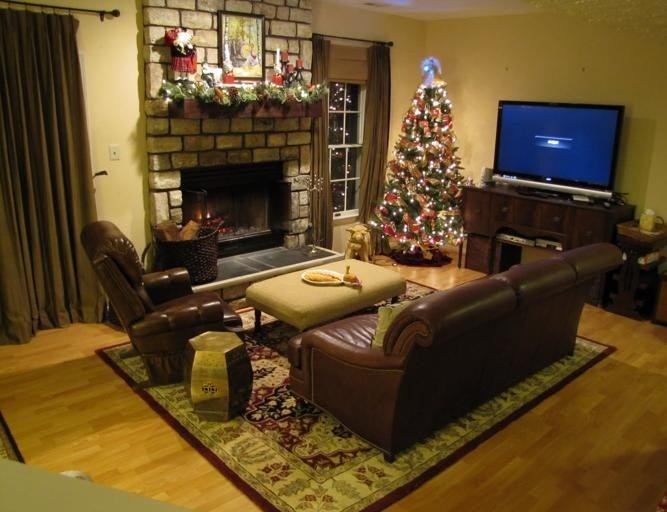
[165,26,197,84]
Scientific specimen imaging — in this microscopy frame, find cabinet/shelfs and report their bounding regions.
[610,220,667,321]
[457,185,636,308]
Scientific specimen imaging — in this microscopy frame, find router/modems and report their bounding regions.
[571,194,590,203]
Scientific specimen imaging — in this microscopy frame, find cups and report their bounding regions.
[638,212,664,232]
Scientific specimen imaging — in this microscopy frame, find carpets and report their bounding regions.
[95,278,618,512]
[0,411,25,464]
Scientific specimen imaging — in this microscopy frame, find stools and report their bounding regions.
[184,329,253,421]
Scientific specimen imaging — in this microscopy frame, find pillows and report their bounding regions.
[370,291,436,349]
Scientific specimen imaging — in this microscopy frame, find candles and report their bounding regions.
[295,59,303,70]
[282,51,288,62]
[286,63,293,75]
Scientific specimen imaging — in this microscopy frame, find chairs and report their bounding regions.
[79,219,245,386]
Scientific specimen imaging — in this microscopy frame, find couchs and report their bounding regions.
[285,241,624,462]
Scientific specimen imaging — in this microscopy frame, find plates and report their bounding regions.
[302,270,362,287]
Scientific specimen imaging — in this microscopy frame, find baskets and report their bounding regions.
[158,226,219,282]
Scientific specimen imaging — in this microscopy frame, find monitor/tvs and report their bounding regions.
[492,99,626,203]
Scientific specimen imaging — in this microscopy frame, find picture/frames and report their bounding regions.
[218,9,266,82]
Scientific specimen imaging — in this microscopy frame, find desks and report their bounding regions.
[189,242,344,302]
